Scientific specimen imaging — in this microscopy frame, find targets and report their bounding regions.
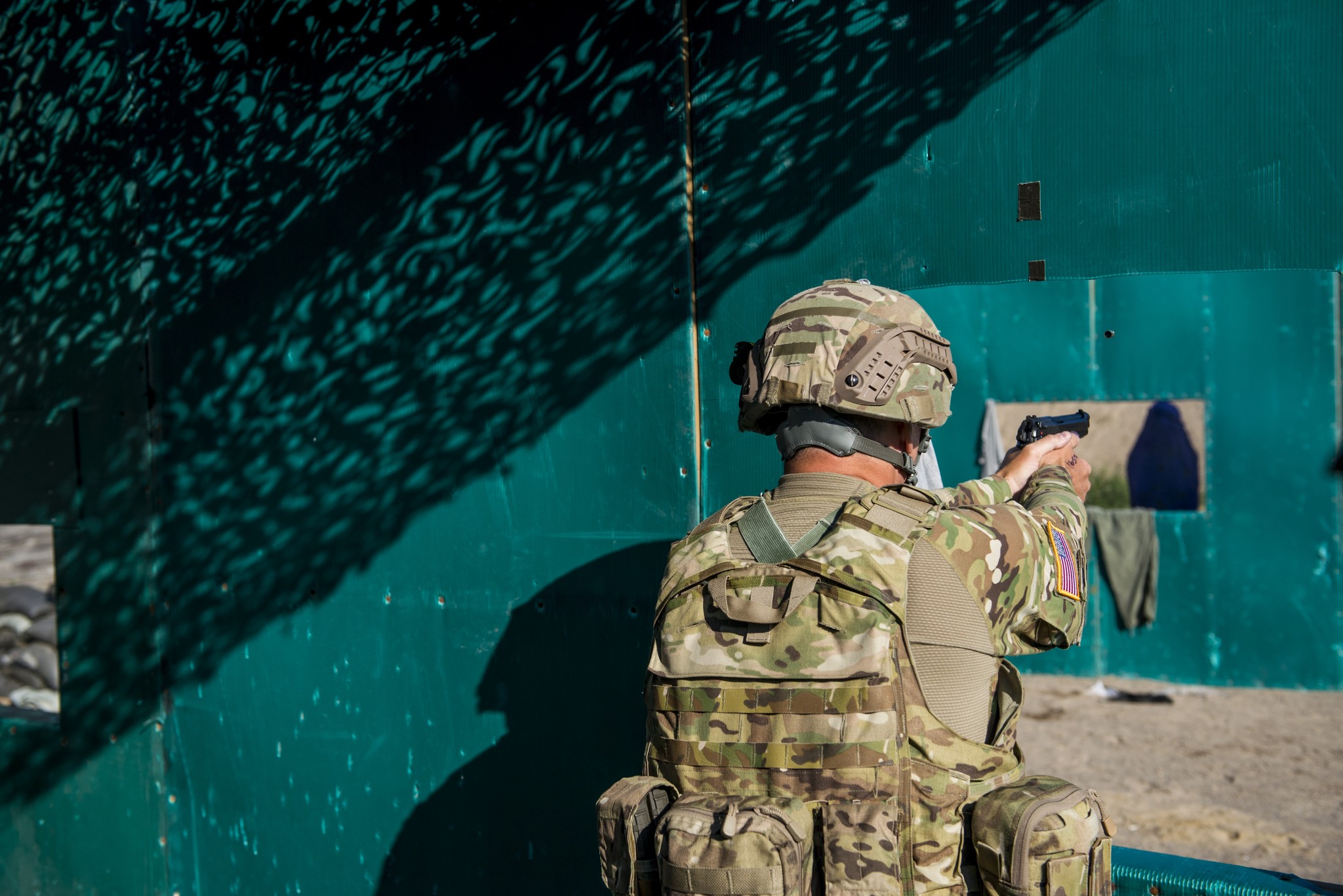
[1124,399,1201,511]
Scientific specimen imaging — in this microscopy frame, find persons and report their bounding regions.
[649,278,1092,896]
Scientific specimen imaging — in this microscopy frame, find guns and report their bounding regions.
[1018,408,1094,456]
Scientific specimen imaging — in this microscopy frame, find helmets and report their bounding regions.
[729,278,958,436]
